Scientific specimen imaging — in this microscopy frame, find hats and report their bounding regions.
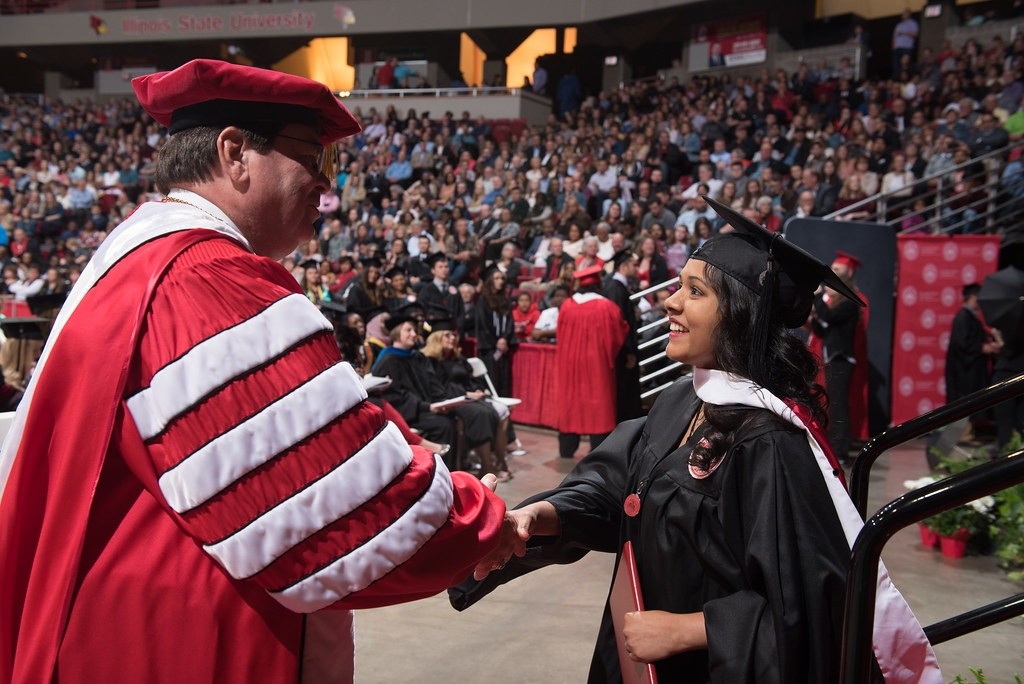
[573,264,605,286]
[692,195,868,388]
[130,57,361,182]
[832,248,863,272]
[298,250,499,330]
[605,245,632,267]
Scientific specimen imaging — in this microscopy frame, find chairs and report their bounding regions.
[468,358,522,447]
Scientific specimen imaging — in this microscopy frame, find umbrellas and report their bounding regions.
[977,267,1024,330]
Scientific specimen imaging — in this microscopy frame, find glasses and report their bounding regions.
[241,126,325,175]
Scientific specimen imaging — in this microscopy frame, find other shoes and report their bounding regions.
[478,465,510,482]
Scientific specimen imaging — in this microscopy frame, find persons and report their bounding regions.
[0,5,1024,480]
[445,198,944,684]
[0,55,530,684]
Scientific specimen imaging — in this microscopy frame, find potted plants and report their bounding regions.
[918,505,980,558]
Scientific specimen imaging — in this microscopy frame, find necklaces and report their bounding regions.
[689,402,703,437]
